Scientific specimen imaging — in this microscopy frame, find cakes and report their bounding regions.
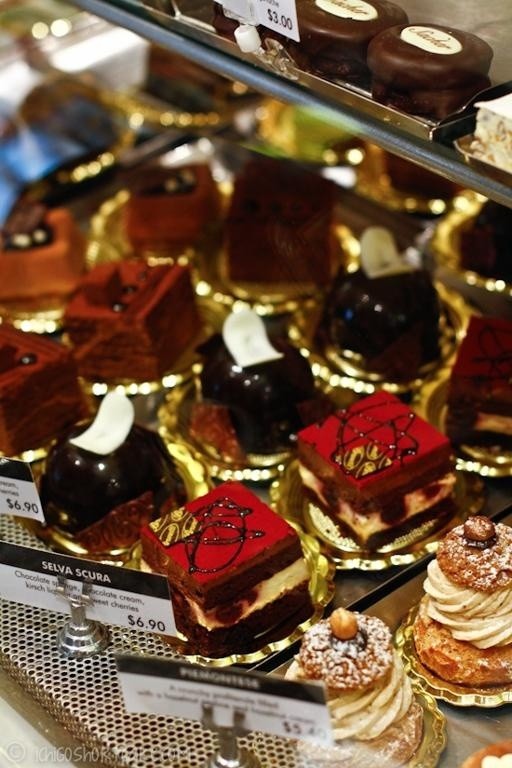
[317,227,445,378]
[0,208,87,314]
[457,190,512,289]
[364,21,494,124]
[289,100,366,168]
[35,388,189,555]
[381,145,466,200]
[296,388,459,552]
[62,259,203,385]
[444,312,512,452]
[278,0,411,81]
[141,38,264,117]
[121,160,225,259]
[0,320,91,459]
[221,156,336,287]
[478,746,512,767]
[411,512,512,692]
[141,476,315,659]
[472,91,511,174]
[186,299,340,470]
[281,606,425,768]
